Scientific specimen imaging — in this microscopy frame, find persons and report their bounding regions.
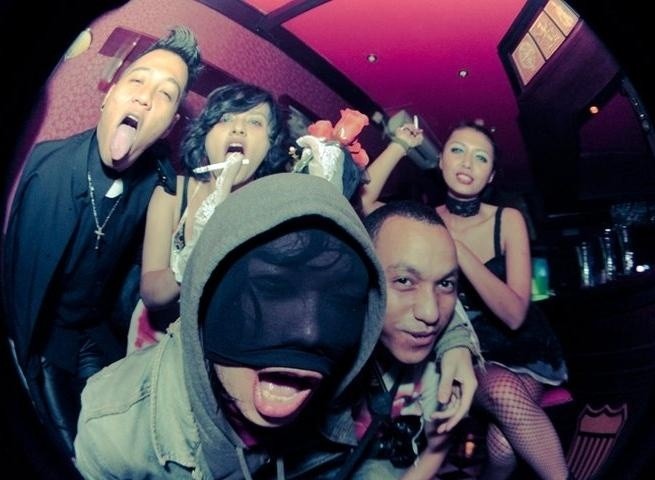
[1,23,574,479]
